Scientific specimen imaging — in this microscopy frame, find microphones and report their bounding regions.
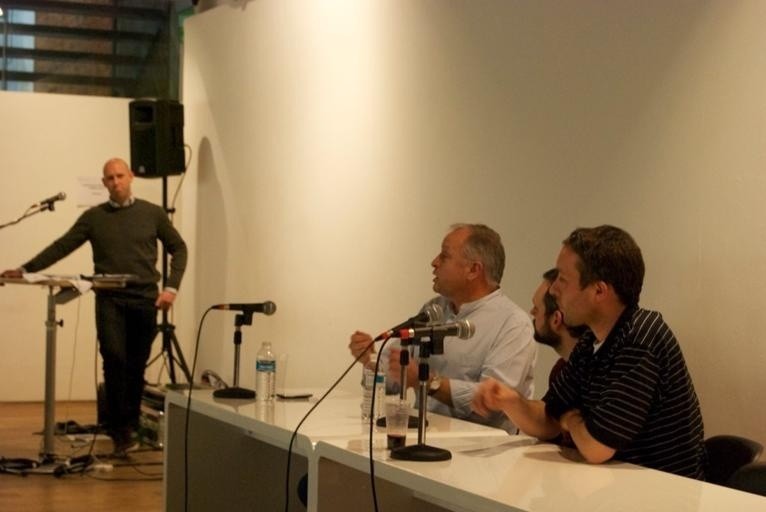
[400,320,474,341]
[374,304,444,343]
[31,191,66,209]
[212,300,276,316]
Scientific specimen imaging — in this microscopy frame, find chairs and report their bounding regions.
[702,433,764,489]
[728,460,764,495]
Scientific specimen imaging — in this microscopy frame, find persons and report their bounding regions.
[472,221,707,484]
[0,157,186,458]
[531,268,590,453]
[353,220,538,442]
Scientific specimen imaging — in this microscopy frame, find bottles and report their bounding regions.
[362,353,387,424]
[254,339,276,402]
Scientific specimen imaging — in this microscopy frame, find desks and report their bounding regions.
[312,430,765,511]
[154,383,510,511]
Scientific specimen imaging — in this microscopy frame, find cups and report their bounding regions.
[385,401,410,450]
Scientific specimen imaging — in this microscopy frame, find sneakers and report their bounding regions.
[112,433,141,455]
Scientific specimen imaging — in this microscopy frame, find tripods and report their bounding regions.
[145,181,194,393]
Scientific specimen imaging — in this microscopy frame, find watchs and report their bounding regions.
[426,375,442,397]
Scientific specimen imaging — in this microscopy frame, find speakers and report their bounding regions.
[129,97,185,178]
